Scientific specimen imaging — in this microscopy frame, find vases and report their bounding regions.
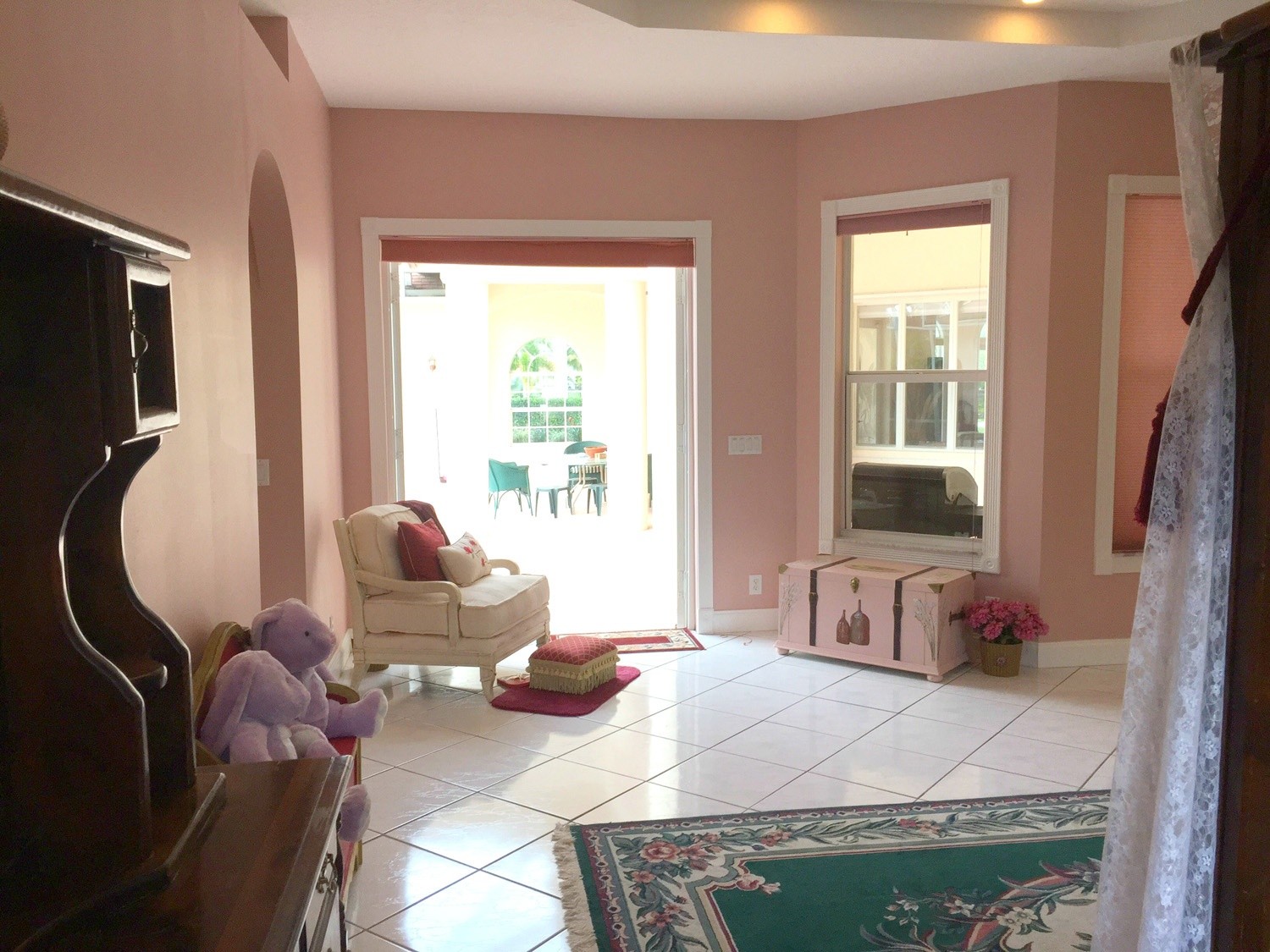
[976,638,1026,679]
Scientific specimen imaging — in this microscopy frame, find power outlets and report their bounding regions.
[748,574,764,597]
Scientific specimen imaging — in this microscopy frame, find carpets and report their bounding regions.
[544,788,1117,952]
[547,627,708,654]
[487,663,642,720]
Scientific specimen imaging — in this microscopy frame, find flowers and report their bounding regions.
[963,597,1047,645]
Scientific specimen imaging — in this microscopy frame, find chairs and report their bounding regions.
[194,620,370,903]
[331,497,553,705]
[484,457,534,521]
[534,438,612,519]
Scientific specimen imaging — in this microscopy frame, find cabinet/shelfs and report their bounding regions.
[773,553,980,690]
[0,168,229,952]
[16,750,360,952]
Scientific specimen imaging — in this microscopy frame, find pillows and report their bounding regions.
[430,531,495,588]
[394,516,448,582]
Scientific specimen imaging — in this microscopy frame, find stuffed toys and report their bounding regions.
[202,598,389,842]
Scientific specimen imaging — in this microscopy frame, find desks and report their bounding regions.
[568,451,608,515]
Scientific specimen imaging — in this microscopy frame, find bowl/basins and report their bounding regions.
[584,447,607,459]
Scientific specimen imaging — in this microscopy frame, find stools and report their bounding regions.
[526,632,619,695]
